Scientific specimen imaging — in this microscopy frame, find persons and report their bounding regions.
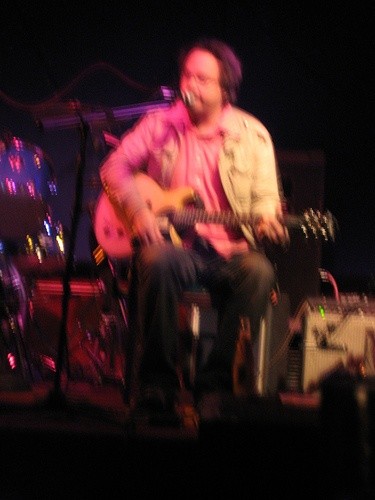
[96,38,290,430]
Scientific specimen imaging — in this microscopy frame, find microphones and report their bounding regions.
[160,87,195,105]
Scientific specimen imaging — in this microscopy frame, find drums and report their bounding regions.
[1,256,35,336]
[1,137,59,236]
[16,220,67,272]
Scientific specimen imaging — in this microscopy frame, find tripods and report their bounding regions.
[2,90,182,423]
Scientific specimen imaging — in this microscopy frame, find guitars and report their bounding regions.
[95,171,333,262]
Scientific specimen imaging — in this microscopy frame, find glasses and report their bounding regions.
[180,68,223,85]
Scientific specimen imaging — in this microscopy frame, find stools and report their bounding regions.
[113,280,275,414]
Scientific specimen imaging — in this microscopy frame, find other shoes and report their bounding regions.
[166,398,200,429]
